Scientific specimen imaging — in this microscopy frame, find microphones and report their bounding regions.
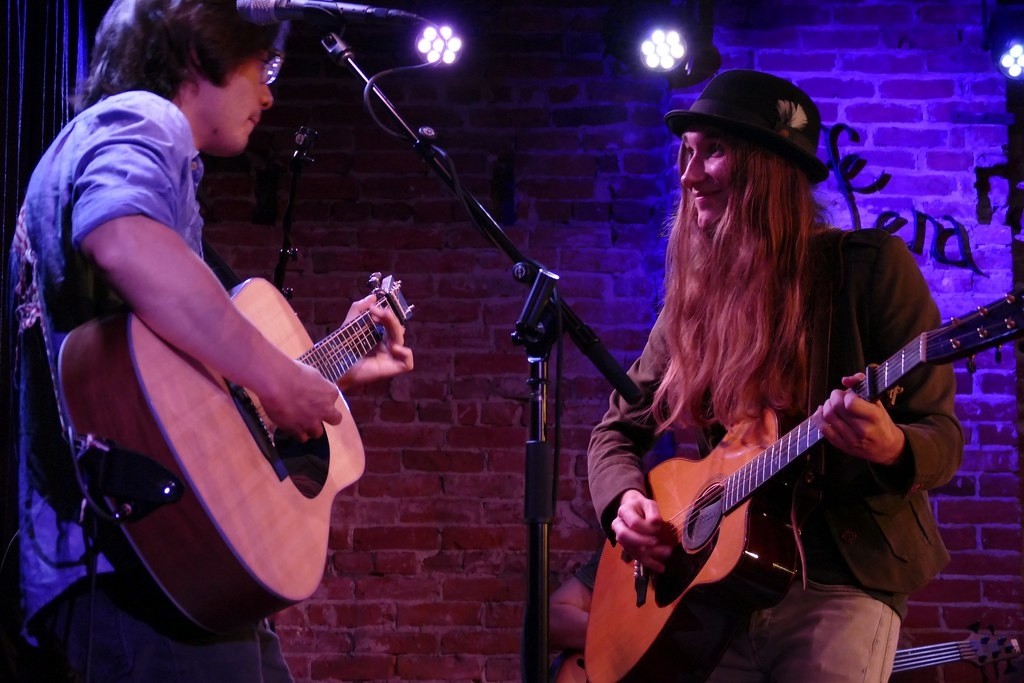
[234,0,400,25]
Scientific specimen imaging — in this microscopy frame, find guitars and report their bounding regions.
[583,290,1023,683]
[56,270,416,636]
[548,623,1022,683]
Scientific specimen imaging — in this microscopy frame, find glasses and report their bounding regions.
[248,53,282,86]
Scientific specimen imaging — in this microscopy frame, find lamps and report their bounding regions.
[632,21,694,75]
[412,21,465,70]
[998,39,1024,79]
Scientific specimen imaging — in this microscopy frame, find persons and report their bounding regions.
[548,535,606,651]
[583,69,969,683]
[9,0,415,681]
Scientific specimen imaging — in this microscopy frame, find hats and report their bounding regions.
[663,70,830,187]
